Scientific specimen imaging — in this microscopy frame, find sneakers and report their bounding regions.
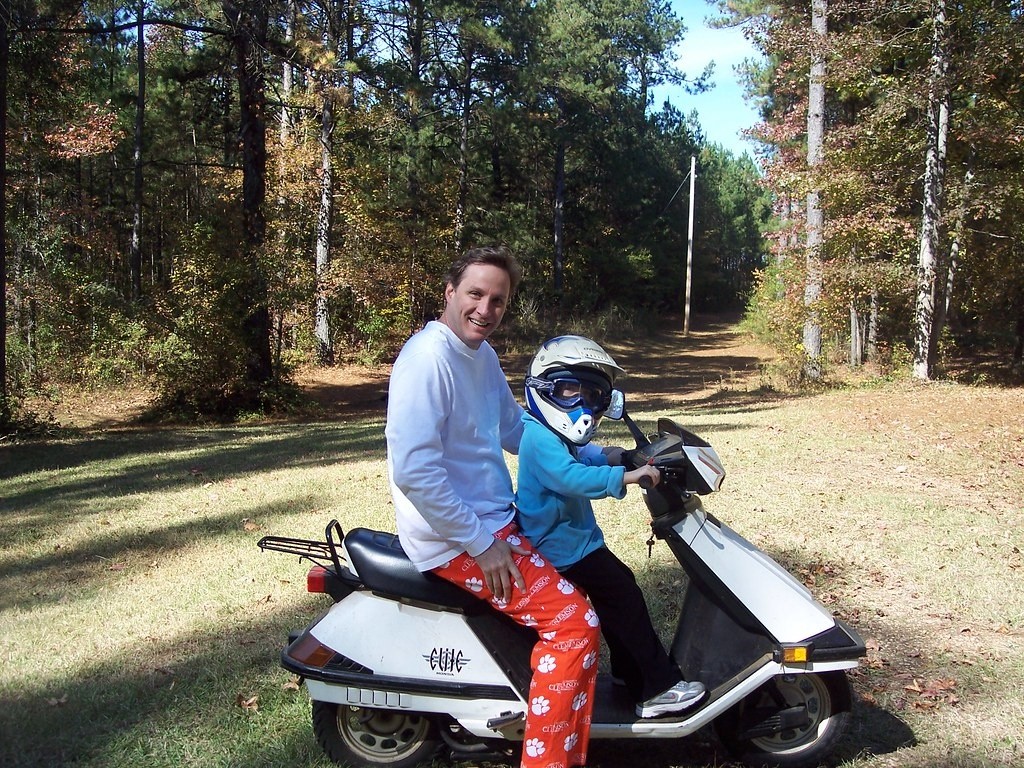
[635,681,706,719]
[610,670,628,686]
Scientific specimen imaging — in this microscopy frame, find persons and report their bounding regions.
[517,335,708,720]
[386,249,628,768]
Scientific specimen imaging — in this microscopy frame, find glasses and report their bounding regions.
[525,375,607,411]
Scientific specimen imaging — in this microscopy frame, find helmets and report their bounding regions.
[524,334,626,448]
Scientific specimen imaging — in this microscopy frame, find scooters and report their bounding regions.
[256,389,867,768]
